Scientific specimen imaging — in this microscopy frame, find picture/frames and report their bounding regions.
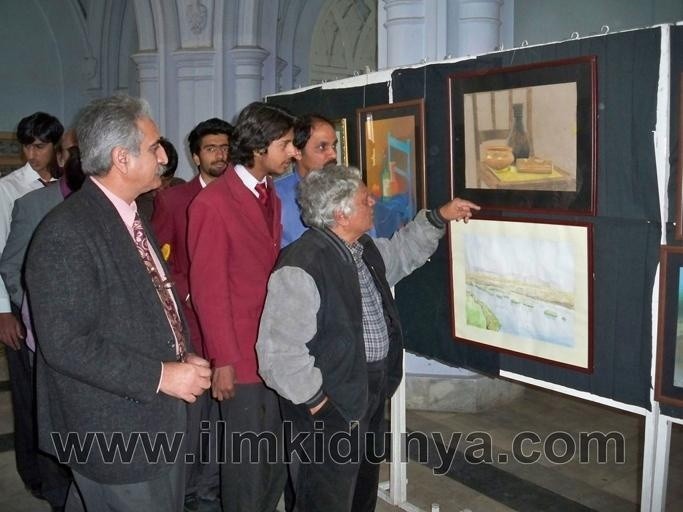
[328,117,348,168]
[447,54,598,217]
[356,99,426,214]
[448,214,593,374]
[654,246,683,407]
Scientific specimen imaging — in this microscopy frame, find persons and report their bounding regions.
[0,93,482,511]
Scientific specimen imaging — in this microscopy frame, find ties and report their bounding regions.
[254,182,273,239]
[132,212,186,363]
